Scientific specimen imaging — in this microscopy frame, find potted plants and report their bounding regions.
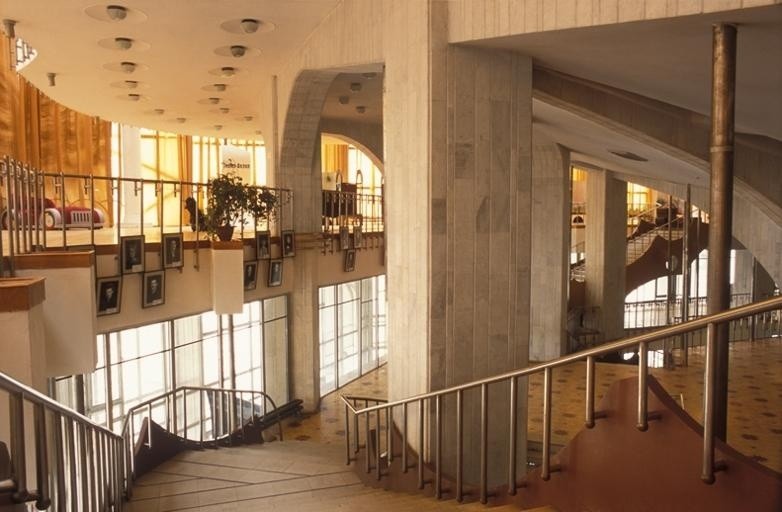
[197,174,281,240]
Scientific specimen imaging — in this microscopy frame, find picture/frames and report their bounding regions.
[120,235,146,276]
[161,232,185,270]
[140,269,165,309]
[96,275,122,317]
[339,225,364,272]
[243,229,296,291]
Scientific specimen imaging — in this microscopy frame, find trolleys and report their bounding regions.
[323,169,363,227]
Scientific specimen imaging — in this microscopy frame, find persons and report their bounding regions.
[184,197,212,231]
